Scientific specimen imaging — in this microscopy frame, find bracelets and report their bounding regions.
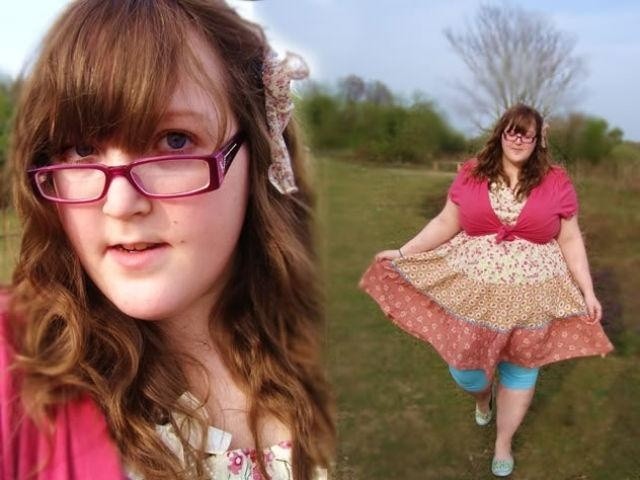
[397,248,405,258]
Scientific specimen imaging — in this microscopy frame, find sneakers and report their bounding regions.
[491,457,514,477]
[475,377,497,426]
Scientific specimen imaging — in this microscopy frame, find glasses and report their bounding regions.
[503,131,537,143]
[27,129,245,204]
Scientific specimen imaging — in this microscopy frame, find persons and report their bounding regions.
[358,101,619,480]
[0,1,342,480]
